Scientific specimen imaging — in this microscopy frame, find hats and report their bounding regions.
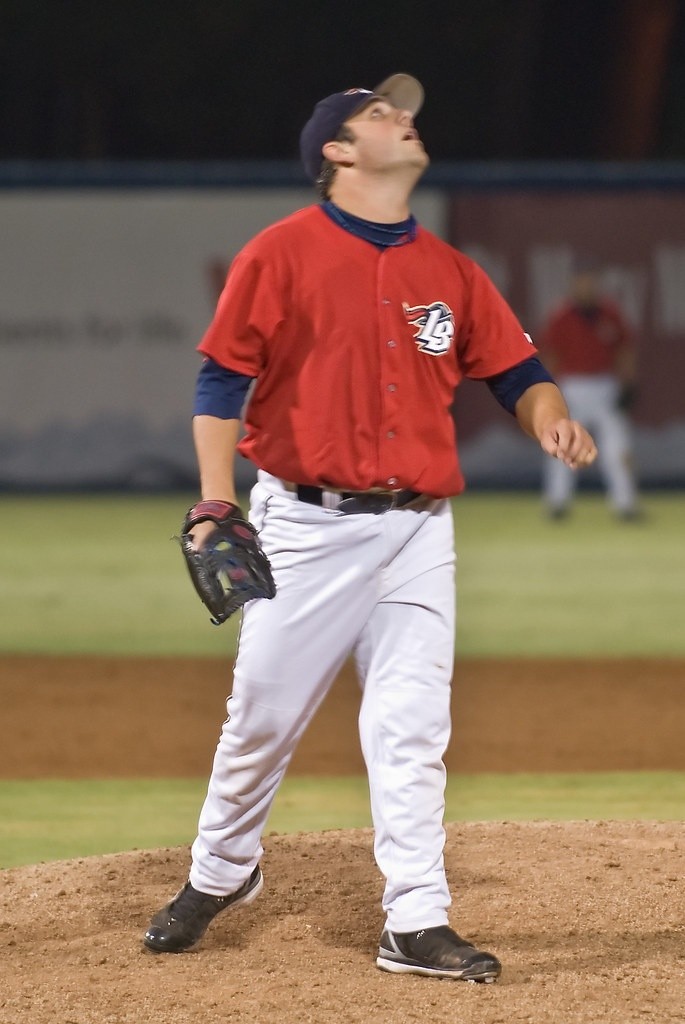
[300,74,425,186]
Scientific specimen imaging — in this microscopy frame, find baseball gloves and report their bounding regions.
[178,499,277,627]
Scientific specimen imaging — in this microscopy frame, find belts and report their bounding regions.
[298,484,423,515]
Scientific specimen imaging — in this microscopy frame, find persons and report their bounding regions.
[143,73,599,981]
[529,268,644,524]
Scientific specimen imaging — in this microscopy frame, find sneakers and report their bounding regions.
[143,863,264,954]
[376,925,501,983]
